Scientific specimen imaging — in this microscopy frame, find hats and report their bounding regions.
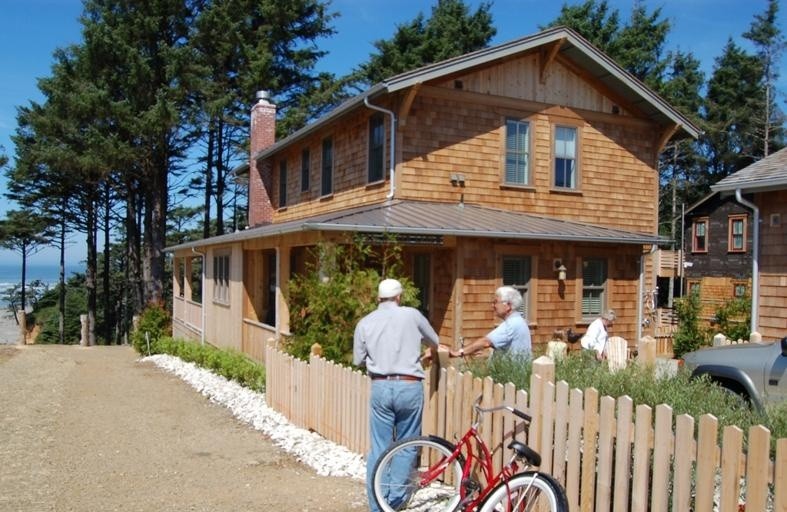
[379,279,401,298]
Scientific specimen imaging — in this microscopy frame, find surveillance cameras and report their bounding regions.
[684,262,694,268]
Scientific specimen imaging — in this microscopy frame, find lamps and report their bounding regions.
[555,257,567,283]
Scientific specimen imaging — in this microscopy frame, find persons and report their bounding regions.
[580,309,616,361]
[440,287,532,369]
[546,328,568,362]
[353,279,439,512]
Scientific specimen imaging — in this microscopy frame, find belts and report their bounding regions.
[373,374,417,380]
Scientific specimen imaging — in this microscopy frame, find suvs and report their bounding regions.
[676,332,786,434]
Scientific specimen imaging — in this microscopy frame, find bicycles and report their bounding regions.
[371,391,570,512]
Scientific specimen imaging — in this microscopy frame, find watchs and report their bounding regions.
[459,349,464,356]
